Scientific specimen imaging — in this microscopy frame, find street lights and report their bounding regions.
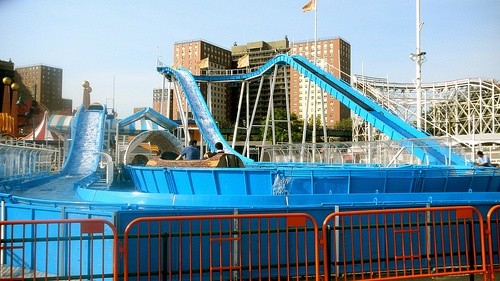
[29,83,40,144]
[409,0,428,132]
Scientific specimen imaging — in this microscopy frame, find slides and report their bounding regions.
[58,104,106,178]
[156,51,471,167]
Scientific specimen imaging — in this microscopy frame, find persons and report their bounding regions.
[320,147,325,158]
[175,139,200,160]
[204,142,224,159]
[474,151,492,167]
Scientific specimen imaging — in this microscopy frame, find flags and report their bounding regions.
[302,0,316,13]
